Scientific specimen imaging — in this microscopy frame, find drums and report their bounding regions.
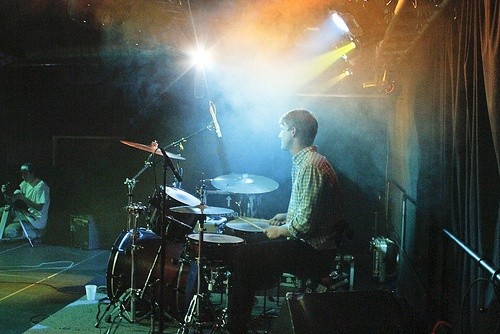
[104,227,210,317]
[145,185,202,243]
[183,232,245,273]
[219,219,283,290]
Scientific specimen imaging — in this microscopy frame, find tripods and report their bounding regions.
[106,154,229,334]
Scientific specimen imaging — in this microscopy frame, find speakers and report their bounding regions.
[70,214,101,250]
[269,287,429,334]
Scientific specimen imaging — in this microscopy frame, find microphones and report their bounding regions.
[161,149,182,182]
[165,215,192,231]
[209,106,222,137]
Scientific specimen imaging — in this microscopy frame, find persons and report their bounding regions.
[225,109,339,334]
[4,162,50,248]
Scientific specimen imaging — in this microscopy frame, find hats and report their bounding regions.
[16,162,35,173]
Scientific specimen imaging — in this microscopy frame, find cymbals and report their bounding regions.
[120,140,187,160]
[211,173,280,194]
[167,204,234,215]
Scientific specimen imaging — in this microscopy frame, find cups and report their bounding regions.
[85,285,97,300]
